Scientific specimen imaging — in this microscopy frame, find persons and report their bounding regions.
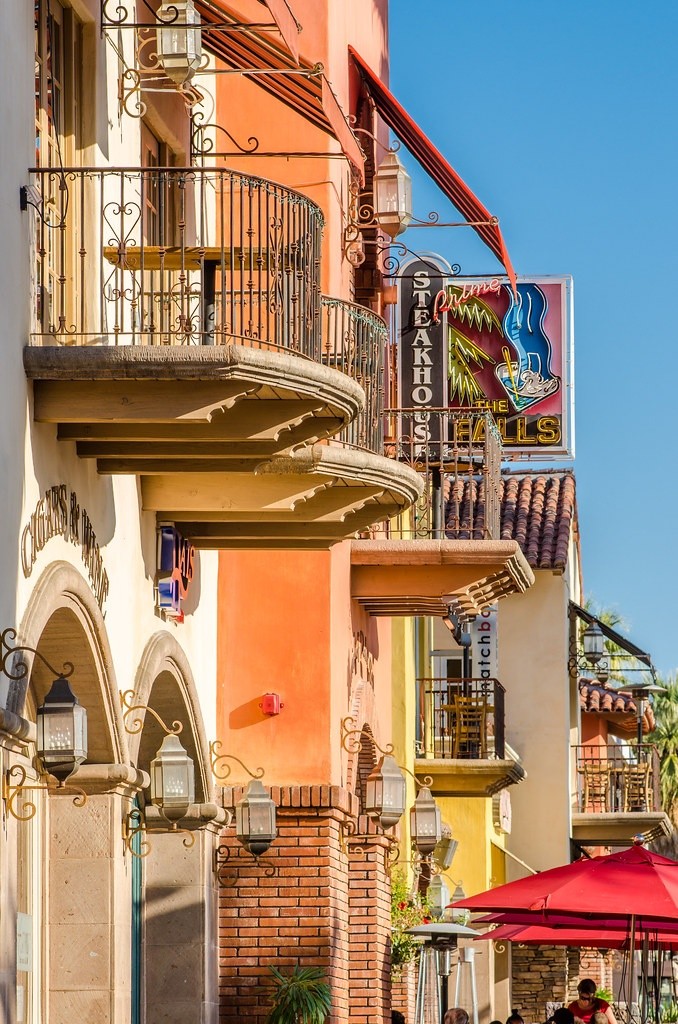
[568,979,617,1024]
[490,1007,608,1024]
[444,1008,469,1024]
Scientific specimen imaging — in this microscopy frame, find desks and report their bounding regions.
[102,242,307,346]
[442,704,494,757]
[578,768,653,811]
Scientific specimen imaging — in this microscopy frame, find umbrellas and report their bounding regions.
[445,834,678,1024]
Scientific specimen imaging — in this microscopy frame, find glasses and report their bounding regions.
[579,994,594,1001]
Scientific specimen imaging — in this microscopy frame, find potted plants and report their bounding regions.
[267,962,331,1024]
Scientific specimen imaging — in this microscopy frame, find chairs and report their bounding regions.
[452,694,487,759]
[582,759,657,813]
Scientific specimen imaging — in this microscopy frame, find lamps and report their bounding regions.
[1,627,86,819]
[119,690,196,858]
[340,718,467,923]
[342,149,415,265]
[567,605,608,678]
[209,741,276,888]
